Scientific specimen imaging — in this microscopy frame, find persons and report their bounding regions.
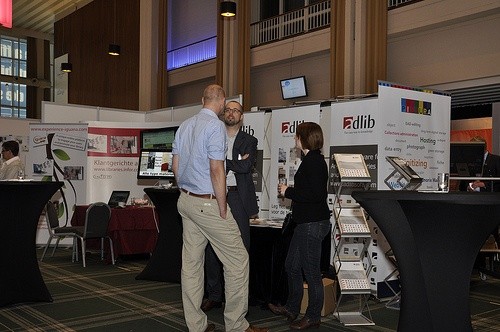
[267,120,332,331]
[457,136,500,267]
[198,99,257,315]
[0,139,24,181]
[171,84,271,332]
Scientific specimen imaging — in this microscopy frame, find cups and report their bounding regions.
[438,173,449,191]
[280,178,287,187]
[18,170,24,180]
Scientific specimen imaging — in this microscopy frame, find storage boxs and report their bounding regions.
[300,277,336,315]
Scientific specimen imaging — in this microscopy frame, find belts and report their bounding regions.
[181,188,216,199]
[228,186,238,192]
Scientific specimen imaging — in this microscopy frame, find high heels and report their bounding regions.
[289,315,321,330]
[269,303,298,322]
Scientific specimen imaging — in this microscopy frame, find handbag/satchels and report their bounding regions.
[281,201,297,237]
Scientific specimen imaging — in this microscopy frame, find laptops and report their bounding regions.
[108,191,130,208]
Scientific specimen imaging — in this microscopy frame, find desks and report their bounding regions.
[246,226,287,309]
[0,178,64,309]
[351,189,500,332]
[71,204,159,265]
[135,187,181,282]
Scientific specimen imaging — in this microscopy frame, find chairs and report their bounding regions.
[40,201,115,267]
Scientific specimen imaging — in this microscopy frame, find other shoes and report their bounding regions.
[200,300,223,313]
[246,325,269,332]
[205,326,215,332]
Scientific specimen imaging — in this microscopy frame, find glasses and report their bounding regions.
[225,108,242,114]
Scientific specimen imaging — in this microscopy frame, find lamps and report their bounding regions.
[61,63,72,73]
[220,1,237,17]
[108,45,120,55]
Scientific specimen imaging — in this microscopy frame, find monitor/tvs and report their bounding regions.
[280,75,308,100]
[137,149,176,179]
[450,142,486,176]
